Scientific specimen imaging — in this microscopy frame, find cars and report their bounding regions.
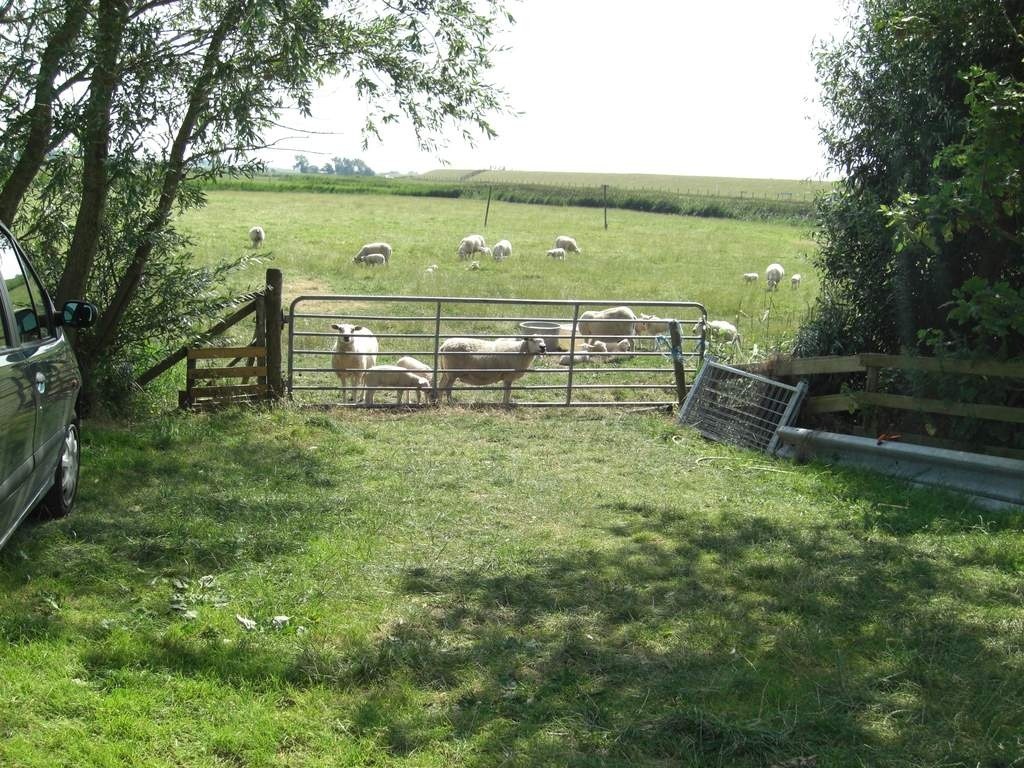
[0,224,97,551]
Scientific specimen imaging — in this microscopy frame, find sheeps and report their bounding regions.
[250,227,265,248]
[743,263,800,291]
[557,304,738,365]
[547,236,581,261]
[425,233,512,273]
[437,334,546,403]
[364,356,437,405]
[331,325,378,402]
[352,243,392,266]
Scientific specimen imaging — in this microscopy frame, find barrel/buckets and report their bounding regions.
[519,322,560,352]
